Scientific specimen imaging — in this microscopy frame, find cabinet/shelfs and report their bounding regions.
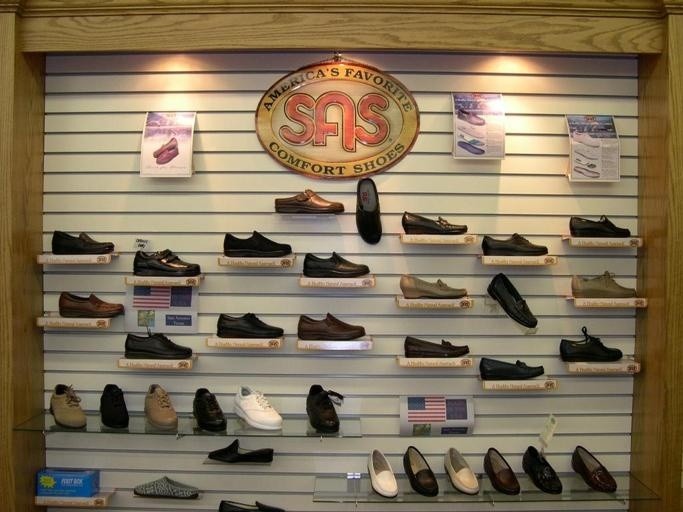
[14,212,661,511]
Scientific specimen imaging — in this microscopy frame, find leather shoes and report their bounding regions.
[570,215,630,238]
[125,332,192,359]
[480,358,544,380]
[402,212,467,234]
[275,189,344,213]
[400,275,467,299]
[219,501,285,512]
[303,252,370,278]
[368,446,562,498]
[208,439,273,463]
[298,313,365,340]
[356,178,381,244]
[482,233,548,255]
[572,446,617,493]
[153,138,178,164]
[572,271,635,298]
[405,337,469,358]
[52,230,114,255]
[306,385,343,434]
[134,249,200,276]
[560,327,622,362]
[573,132,600,146]
[59,293,124,317]
[458,109,485,125]
[224,231,291,257]
[217,313,283,338]
[487,273,537,328]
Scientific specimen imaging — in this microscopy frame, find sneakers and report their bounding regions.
[49,384,283,431]
[134,477,198,499]
